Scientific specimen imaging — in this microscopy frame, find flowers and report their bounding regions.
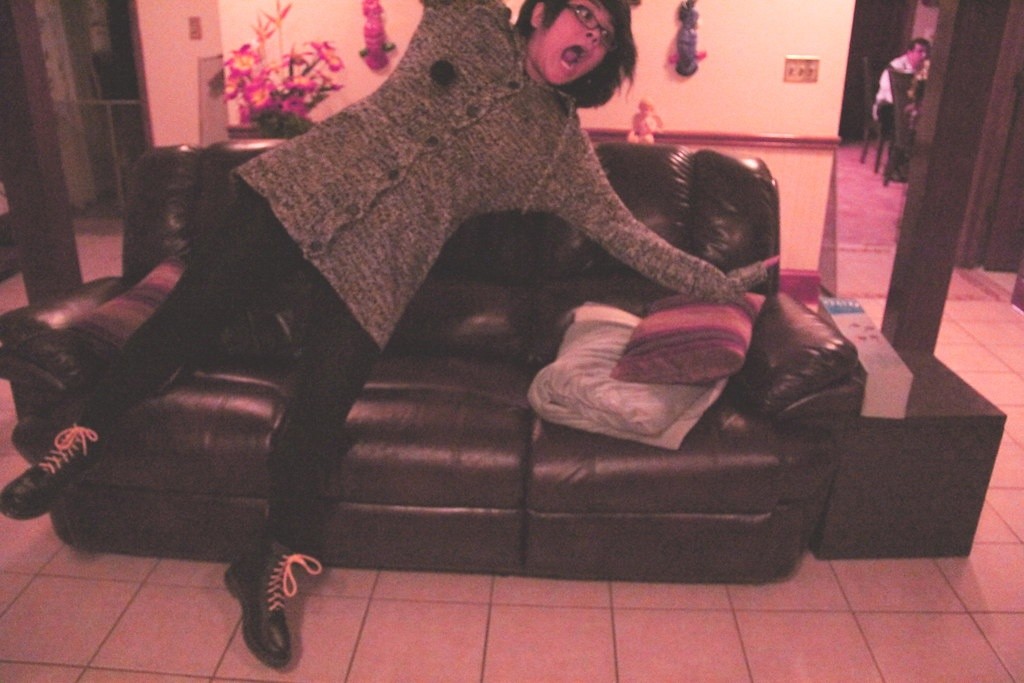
[221,1,346,139]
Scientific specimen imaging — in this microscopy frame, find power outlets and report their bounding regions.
[784,60,819,83]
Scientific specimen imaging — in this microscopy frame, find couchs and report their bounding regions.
[0,140,869,584]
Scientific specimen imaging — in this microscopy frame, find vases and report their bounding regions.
[225,126,268,140]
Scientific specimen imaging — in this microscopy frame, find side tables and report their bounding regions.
[808,348,1008,558]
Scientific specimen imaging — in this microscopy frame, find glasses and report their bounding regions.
[562,1,619,52]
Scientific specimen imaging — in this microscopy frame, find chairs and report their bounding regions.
[883,63,917,185]
[859,55,903,174]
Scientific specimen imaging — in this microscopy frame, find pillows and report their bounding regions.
[611,291,765,385]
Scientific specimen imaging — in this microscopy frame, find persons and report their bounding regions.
[873,38,930,137]
[0,0,780,670]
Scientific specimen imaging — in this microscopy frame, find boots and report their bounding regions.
[224,534,322,667]
[0,421,107,521]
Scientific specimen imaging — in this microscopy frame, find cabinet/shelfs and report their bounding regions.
[582,130,843,306]
